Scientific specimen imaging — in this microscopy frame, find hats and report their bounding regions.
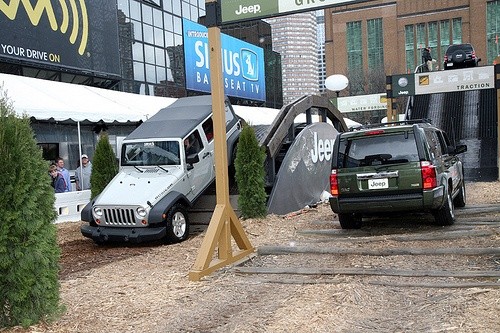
[82,154,88,158]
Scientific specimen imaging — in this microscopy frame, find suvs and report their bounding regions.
[329,118,466,228]
[443,43,482,70]
[81,95,243,245]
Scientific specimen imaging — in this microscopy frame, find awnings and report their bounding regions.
[231,105,362,128]
[0,72,178,124]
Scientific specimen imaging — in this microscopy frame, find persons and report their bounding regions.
[50,165,66,194]
[424,48,433,72]
[55,156,72,192]
[184,138,196,163]
[75,154,93,192]
